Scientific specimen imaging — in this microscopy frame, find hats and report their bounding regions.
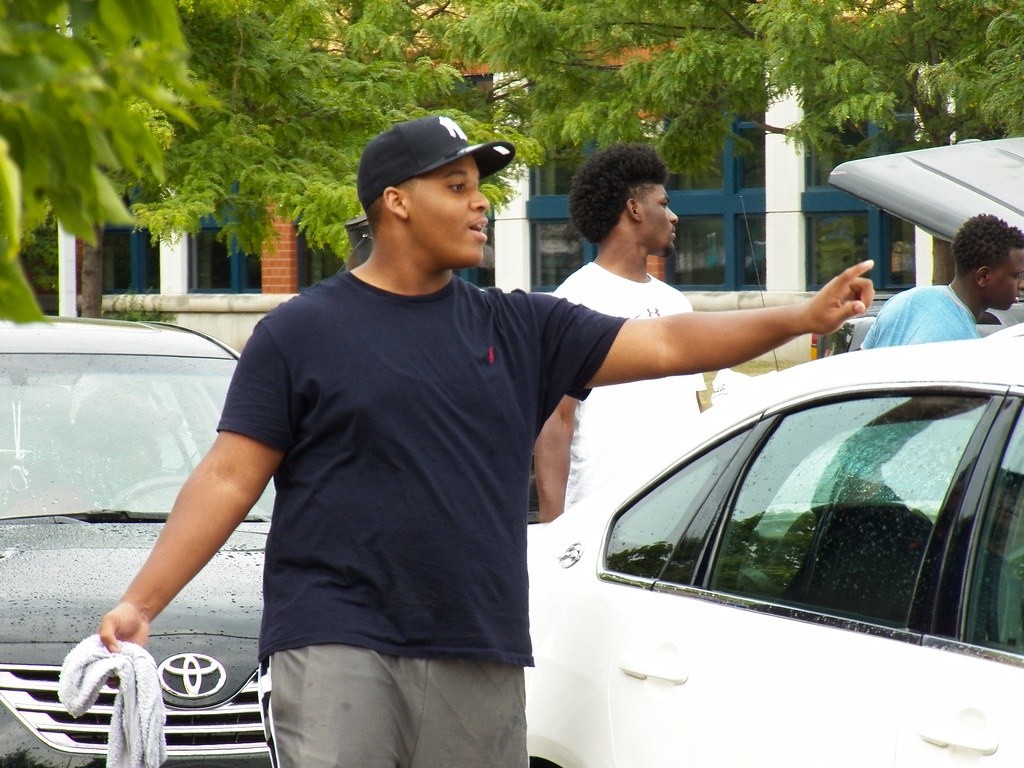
[356,116,515,213]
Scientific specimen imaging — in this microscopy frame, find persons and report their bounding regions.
[779,396,991,630]
[532,144,709,524]
[860,214,1024,351]
[94,115,875,768]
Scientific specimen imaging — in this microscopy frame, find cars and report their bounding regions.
[810,135,1024,359]
[525,321,1023,768]
[0,319,274,768]
